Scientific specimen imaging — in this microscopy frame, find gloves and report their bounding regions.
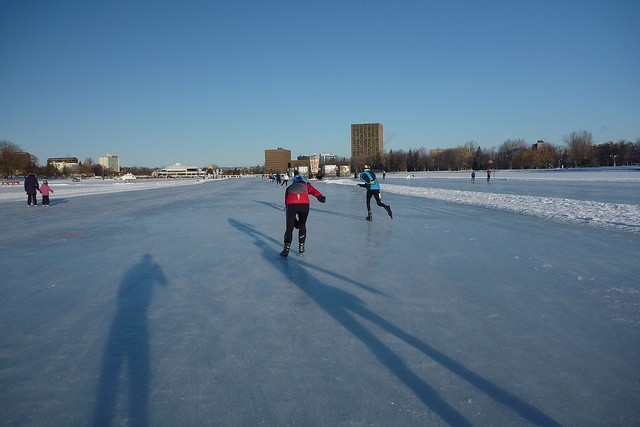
[318,195,326,203]
[294,219,301,228]
[370,180,375,185]
[52,190,54,193]
[356,184,360,189]
[40,191,42,193]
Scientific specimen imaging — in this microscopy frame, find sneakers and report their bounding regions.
[34,205,38,206]
[366,215,372,220]
[27,205,32,206]
[299,247,305,253]
[279,251,288,257]
[46,204,49,206]
[42,204,44,207]
[387,206,391,216]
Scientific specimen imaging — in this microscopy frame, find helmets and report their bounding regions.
[44,181,47,184]
[364,164,370,169]
[292,174,308,183]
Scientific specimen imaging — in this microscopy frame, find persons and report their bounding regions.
[383,170,386,179]
[279,175,326,257]
[357,164,393,222]
[470,172,475,181]
[40,181,53,206]
[267,172,289,186]
[486,168,492,181]
[24,173,39,206]
